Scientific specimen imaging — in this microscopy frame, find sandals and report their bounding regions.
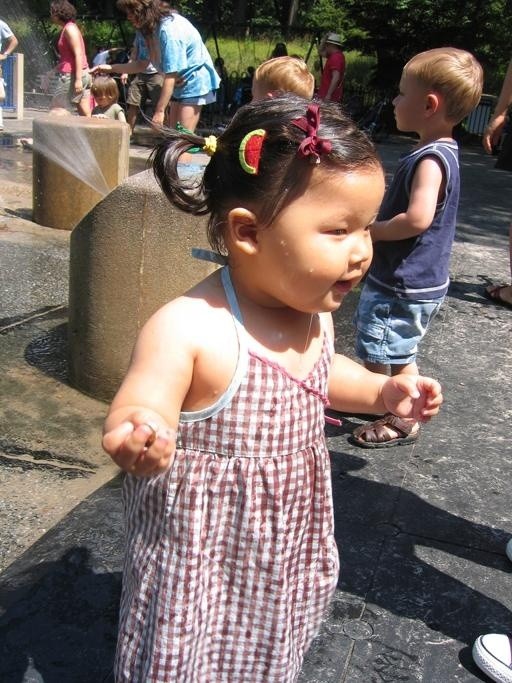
[484,285,512,310]
[353,412,420,448]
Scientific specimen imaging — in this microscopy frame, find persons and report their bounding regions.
[481,54,512,306]
[97,92,447,683]
[120,26,167,144]
[250,55,316,105]
[345,46,485,448]
[122,44,134,109]
[313,30,348,105]
[90,45,126,77]
[271,41,288,58]
[88,0,222,135]
[91,74,127,124]
[0,18,20,133]
[46,0,93,116]
[213,56,229,132]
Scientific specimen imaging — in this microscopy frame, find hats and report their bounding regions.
[326,33,343,46]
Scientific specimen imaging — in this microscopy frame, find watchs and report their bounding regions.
[2,51,9,56]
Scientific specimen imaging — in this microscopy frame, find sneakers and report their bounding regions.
[472,633,512,683]
